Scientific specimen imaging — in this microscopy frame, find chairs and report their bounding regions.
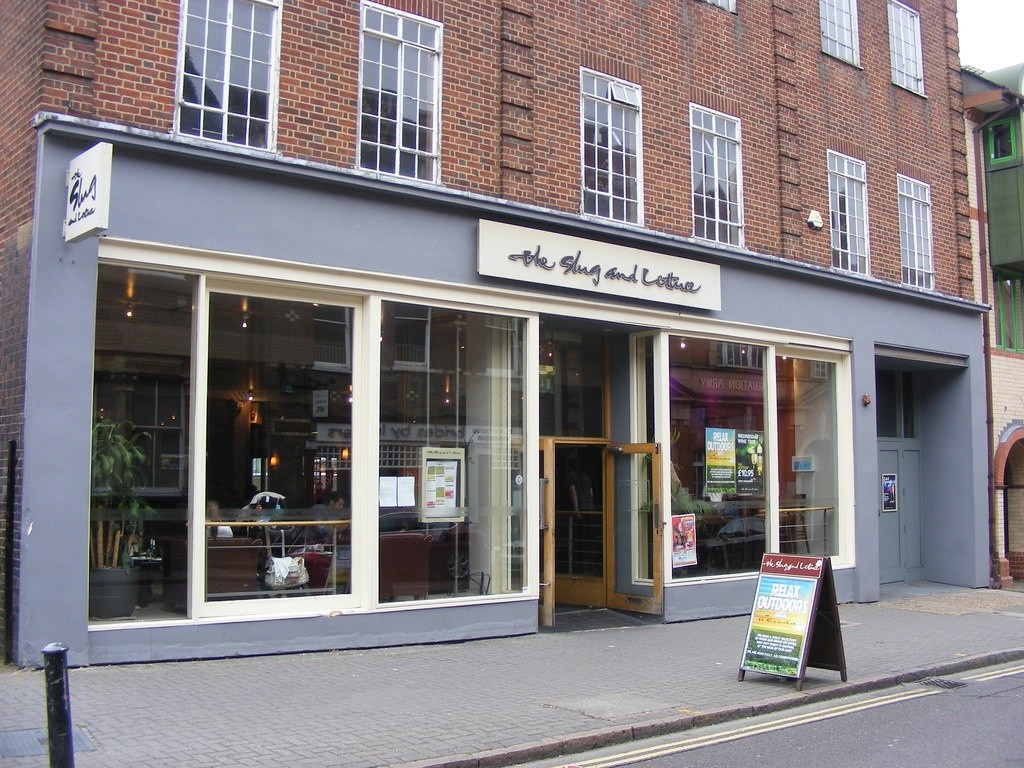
[695,493,810,576]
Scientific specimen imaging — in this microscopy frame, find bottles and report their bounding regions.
[153,539,156,559]
[146,539,154,558]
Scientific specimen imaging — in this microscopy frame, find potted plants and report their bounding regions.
[88,407,148,619]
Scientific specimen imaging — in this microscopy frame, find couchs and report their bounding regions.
[157,533,262,614]
[379,521,469,604]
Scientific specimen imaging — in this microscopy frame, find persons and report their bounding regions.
[580,466,753,539]
[555,455,581,562]
[205,485,349,539]
